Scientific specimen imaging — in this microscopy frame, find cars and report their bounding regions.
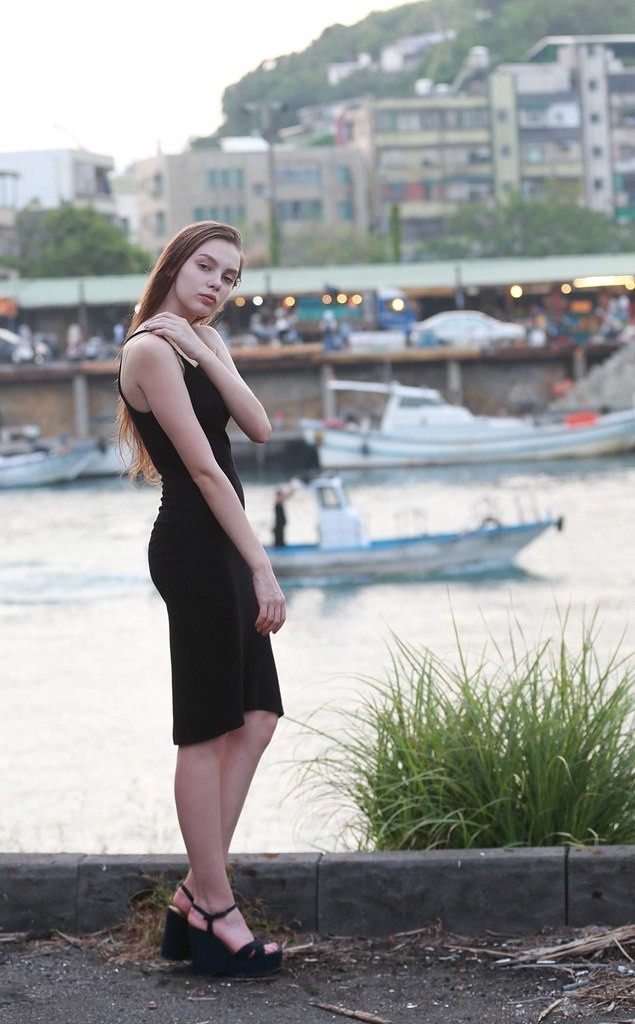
[413,312,527,345]
[0,328,52,364]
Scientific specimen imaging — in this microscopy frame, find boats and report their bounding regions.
[0,426,108,488]
[262,478,563,587]
[299,382,635,470]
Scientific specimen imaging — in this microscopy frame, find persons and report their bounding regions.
[273,488,296,546]
[112,220,287,978]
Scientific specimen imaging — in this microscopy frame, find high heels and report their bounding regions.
[161,883,194,961]
[187,903,282,977]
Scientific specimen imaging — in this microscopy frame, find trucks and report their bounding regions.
[296,285,417,343]
